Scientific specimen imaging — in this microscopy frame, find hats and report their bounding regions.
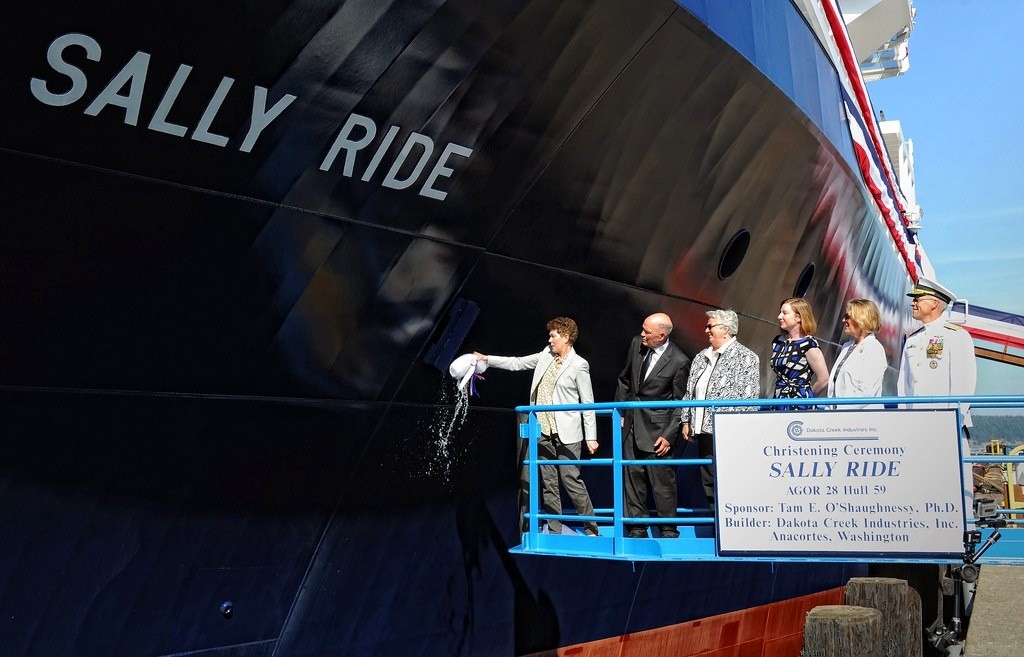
[906,276,957,304]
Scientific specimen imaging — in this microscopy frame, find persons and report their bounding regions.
[824,299,888,409]
[614,313,692,538]
[472,317,601,537]
[681,310,760,538]
[896,277,977,532]
[768,297,830,412]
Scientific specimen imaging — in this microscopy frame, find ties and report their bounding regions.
[639,348,655,384]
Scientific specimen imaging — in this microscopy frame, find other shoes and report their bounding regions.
[628,532,649,538]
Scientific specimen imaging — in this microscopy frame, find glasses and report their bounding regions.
[913,296,940,304]
[705,324,723,330]
[844,313,851,320]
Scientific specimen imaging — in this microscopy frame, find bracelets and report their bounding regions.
[682,422,690,425]
